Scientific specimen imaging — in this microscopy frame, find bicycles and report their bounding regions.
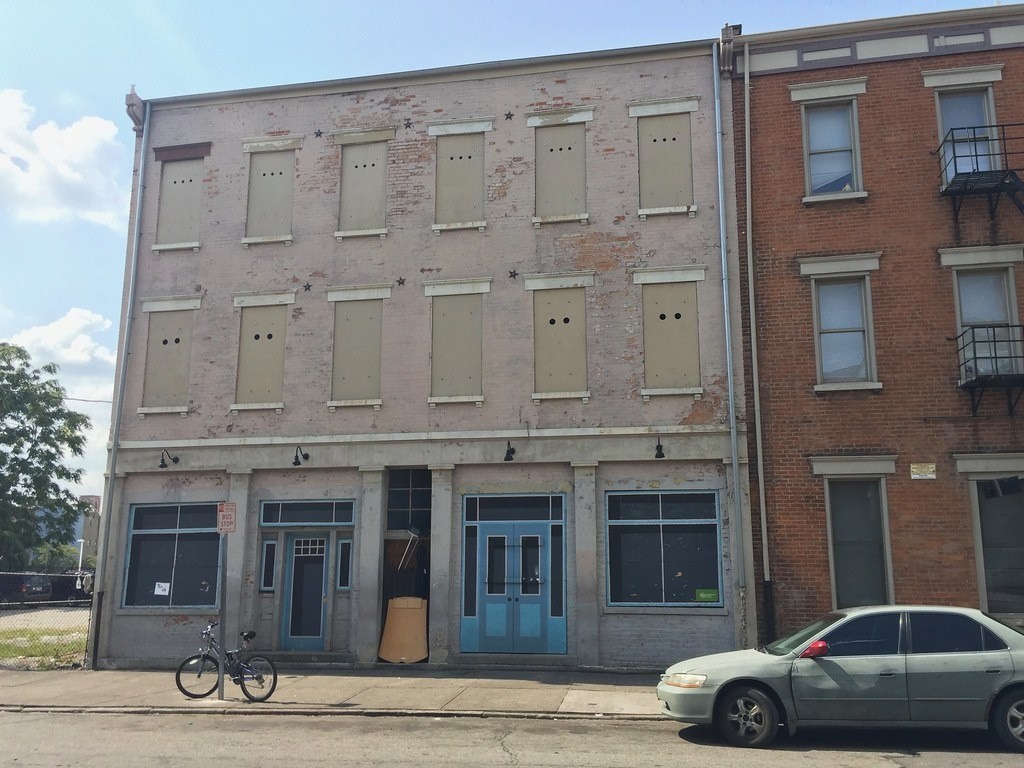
[174,620,278,703]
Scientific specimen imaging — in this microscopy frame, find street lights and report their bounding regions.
[76,538,85,590]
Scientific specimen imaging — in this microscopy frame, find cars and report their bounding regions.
[655,601,1024,755]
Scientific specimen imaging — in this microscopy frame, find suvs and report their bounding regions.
[0,568,53,610]
